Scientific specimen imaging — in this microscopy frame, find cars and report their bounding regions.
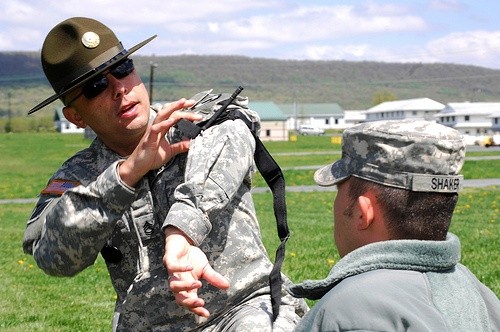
[299,125,327,137]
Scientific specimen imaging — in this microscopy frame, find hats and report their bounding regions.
[313,119,466,195]
[27,16,157,115]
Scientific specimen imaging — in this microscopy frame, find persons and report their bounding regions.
[22,17,310,332]
[294,119,500,332]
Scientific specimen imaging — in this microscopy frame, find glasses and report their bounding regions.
[66,59,135,111]
